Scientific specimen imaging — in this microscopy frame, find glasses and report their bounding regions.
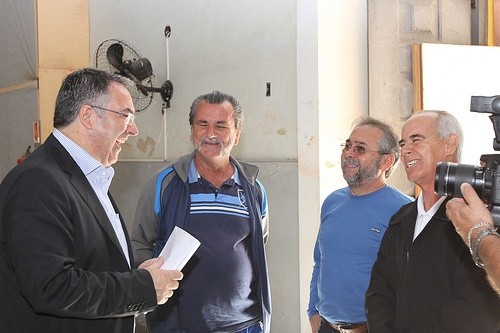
[91,106,134,125]
[192,119,233,132]
[340,143,379,156]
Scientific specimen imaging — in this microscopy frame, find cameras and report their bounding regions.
[434,96,500,228]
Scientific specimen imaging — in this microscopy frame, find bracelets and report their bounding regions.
[472,228,500,268]
[468,222,495,260]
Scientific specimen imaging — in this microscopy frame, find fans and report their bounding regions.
[96,39,173,112]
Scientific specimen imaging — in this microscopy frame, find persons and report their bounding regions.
[130,92,272,333]
[307,115,416,333]
[364,111,500,333]
[0,67,183,333]
[446,183,500,295]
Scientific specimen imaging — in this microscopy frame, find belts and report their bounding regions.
[331,324,368,333]
